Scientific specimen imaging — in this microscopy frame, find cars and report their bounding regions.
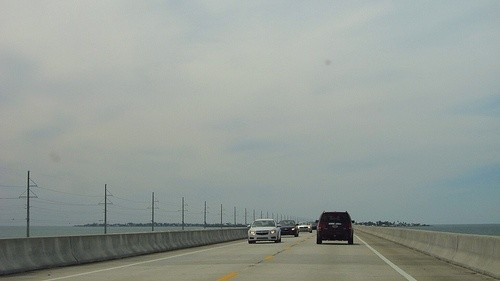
[247,219,282,244]
[278,220,299,237]
[311,222,317,230]
[297,221,312,233]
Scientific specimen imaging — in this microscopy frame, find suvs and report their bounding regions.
[315,210,355,245]
